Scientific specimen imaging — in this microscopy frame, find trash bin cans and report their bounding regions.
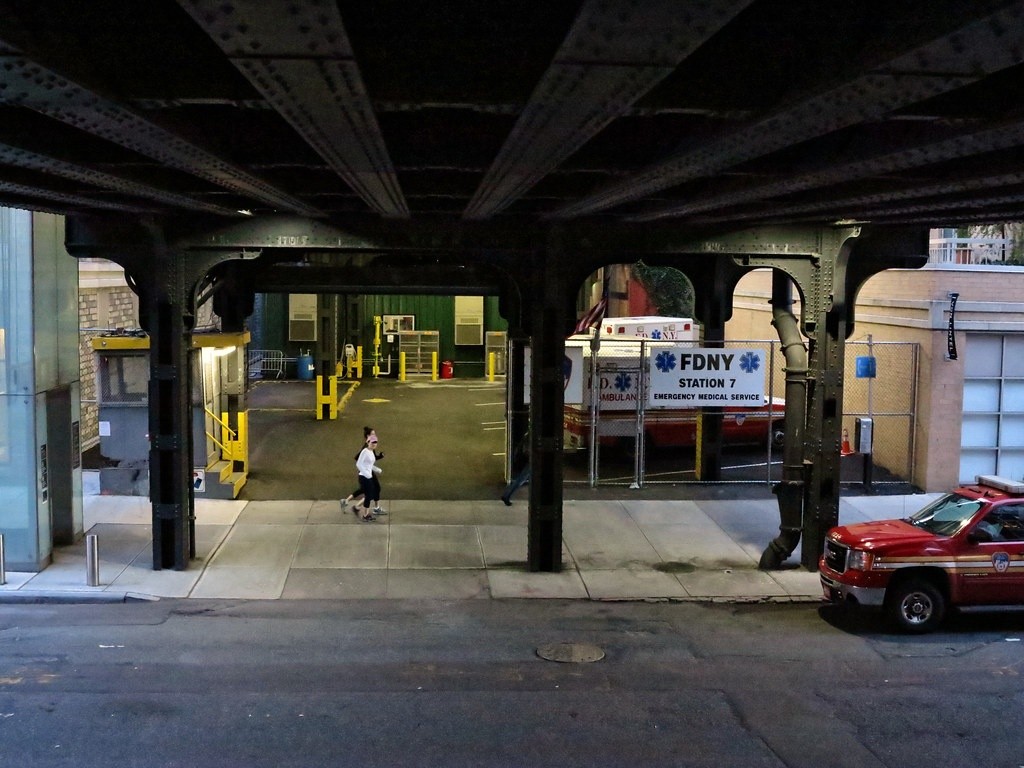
[297,354,314,380]
[440,360,454,379]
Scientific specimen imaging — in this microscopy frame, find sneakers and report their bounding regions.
[351,505,361,518]
[340,499,348,514]
[362,514,376,522]
[372,506,389,515]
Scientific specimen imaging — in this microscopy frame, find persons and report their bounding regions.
[340,427,388,523]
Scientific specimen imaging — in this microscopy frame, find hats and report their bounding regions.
[366,435,378,443]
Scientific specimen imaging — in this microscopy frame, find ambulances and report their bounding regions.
[563,345,787,454]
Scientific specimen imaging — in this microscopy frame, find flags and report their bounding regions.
[572,287,609,334]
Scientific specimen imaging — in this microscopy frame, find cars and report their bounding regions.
[818,485,1024,624]
